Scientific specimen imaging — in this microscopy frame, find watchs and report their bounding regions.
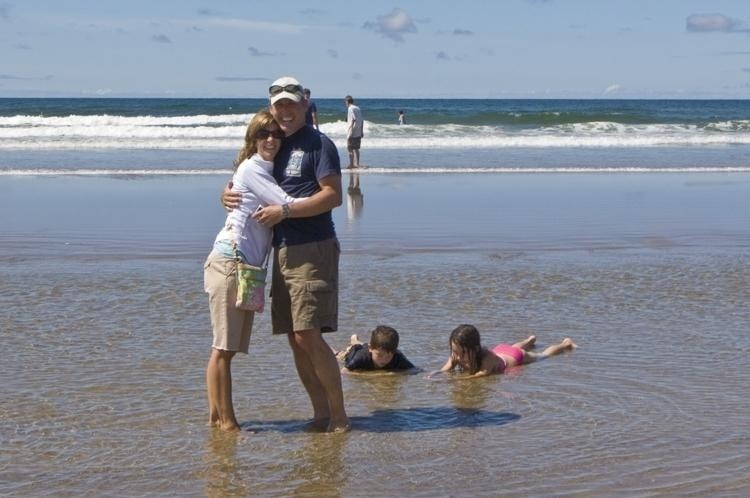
[280,201,292,220]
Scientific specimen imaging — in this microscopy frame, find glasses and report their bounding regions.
[270,84,304,95]
[256,129,285,139]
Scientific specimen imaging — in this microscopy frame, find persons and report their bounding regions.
[427,323,585,382]
[326,323,422,377]
[343,96,364,169]
[203,104,316,437]
[220,76,354,437]
[344,168,364,234]
[398,110,406,125]
[301,86,320,133]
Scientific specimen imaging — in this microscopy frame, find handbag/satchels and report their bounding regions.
[236,262,266,312]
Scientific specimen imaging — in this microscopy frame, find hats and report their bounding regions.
[269,77,304,105]
